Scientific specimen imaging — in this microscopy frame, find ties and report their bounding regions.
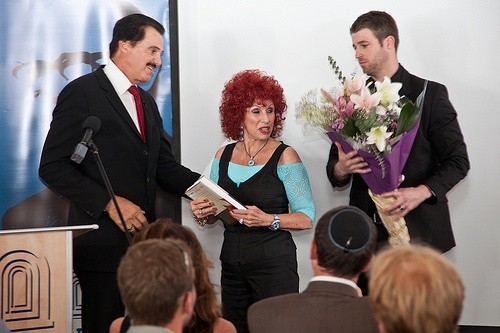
[127,85,147,143]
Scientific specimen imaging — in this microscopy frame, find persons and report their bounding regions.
[116,237,197,333]
[131,218,236,333]
[247,205,380,332]
[189,69,316,333]
[39,13,205,333]
[367,246,466,333]
[325,11,471,298]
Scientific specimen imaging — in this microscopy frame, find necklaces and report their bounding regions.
[243,139,269,166]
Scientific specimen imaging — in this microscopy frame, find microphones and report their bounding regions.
[70,115,102,164]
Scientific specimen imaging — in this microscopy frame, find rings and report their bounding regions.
[127,225,134,230]
[238,218,243,225]
[199,209,203,215]
[399,206,404,211]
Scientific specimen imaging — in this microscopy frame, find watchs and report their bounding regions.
[269,213,280,231]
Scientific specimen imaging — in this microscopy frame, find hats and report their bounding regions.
[327,209,371,252]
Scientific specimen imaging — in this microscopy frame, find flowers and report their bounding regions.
[295,55,429,246]
